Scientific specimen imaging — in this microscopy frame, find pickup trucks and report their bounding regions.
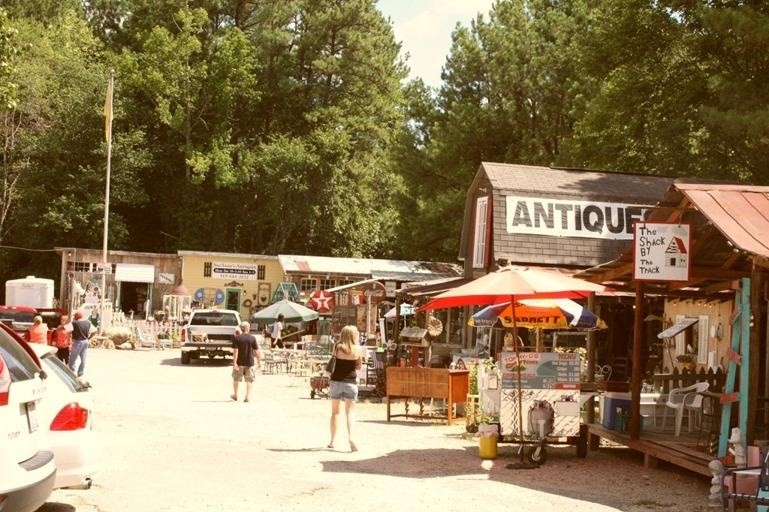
[181,309,246,365]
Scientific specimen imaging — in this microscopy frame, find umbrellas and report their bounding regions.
[253,298,319,330]
[416,267,615,463]
[384,303,416,328]
[467,298,609,351]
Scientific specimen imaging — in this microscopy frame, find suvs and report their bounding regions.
[0,319,59,512]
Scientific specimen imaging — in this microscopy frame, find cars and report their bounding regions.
[27,338,94,489]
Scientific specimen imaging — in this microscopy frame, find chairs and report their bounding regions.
[263,354,278,374]
[661,382,710,438]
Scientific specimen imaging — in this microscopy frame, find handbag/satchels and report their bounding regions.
[326,342,339,373]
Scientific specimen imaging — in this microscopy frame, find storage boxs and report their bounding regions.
[599,392,632,430]
[639,393,660,427]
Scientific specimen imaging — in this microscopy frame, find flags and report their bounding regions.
[104,79,113,144]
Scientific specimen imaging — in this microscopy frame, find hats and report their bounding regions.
[73,311,83,318]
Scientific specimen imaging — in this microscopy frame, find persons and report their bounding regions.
[231,321,260,402]
[88,310,100,328]
[57,316,74,364]
[69,312,90,377]
[328,325,361,451]
[271,313,284,348]
[25,315,48,345]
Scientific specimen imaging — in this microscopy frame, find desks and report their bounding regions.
[386,368,470,426]
